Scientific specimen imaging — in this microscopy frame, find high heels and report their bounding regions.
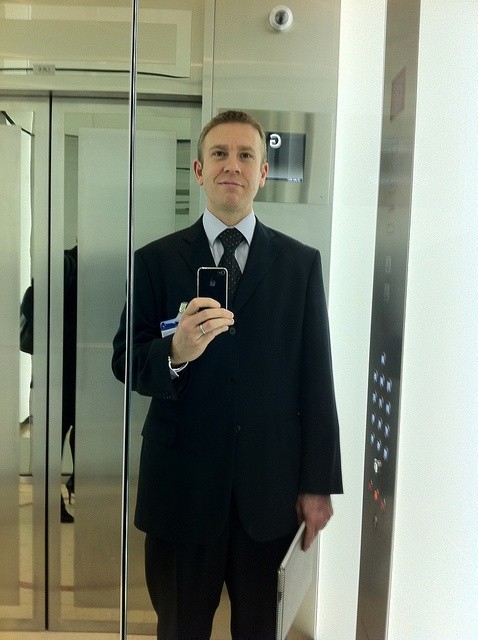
[66,478,74,504]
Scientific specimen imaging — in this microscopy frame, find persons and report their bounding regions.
[110,111,342,639]
[61,243,76,524]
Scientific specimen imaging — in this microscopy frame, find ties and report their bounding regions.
[216,227,247,301]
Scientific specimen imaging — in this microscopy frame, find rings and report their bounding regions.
[199,324,206,335]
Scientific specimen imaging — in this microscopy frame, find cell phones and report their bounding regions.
[196,265,228,326]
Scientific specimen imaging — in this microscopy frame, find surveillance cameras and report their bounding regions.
[269,5,293,30]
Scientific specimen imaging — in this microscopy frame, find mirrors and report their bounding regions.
[0,1,472,640]
[1,92,196,632]
